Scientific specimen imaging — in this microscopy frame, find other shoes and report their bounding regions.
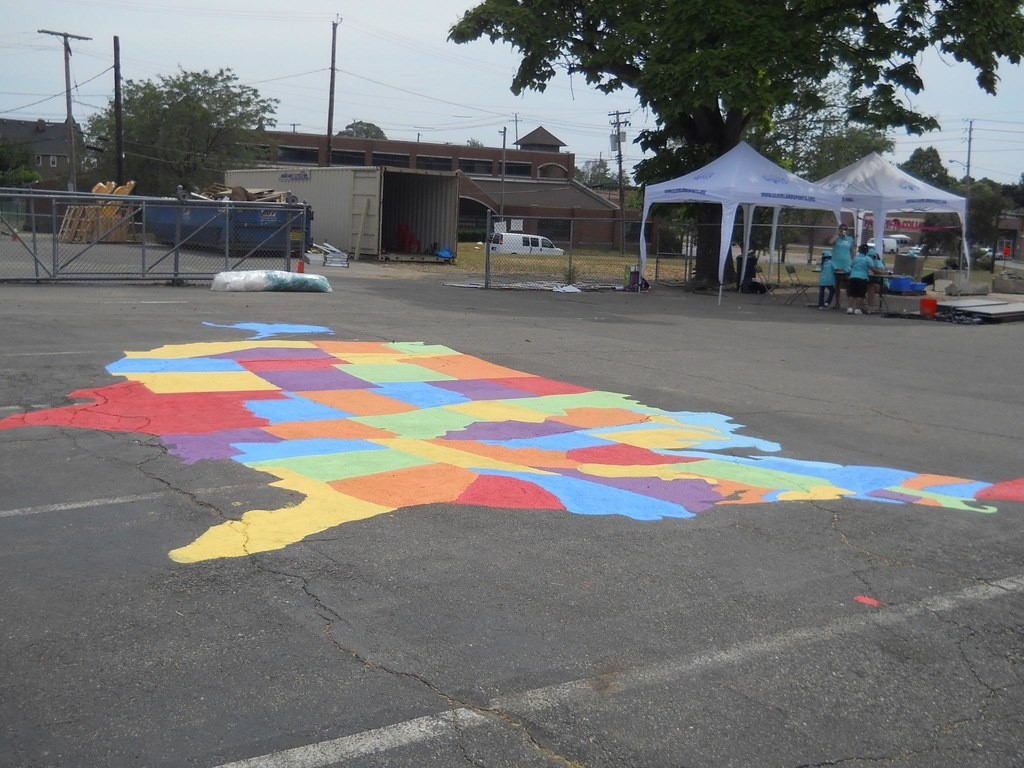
[854,309,862,315]
[844,308,854,313]
[819,306,830,311]
[824,302,828,307]
[867,307,873,314]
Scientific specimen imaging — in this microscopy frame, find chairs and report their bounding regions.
[754,263,810,305]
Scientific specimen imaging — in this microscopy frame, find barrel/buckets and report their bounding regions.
[889,278,897,289]
[920,298,937,318]
[898,277,913,290]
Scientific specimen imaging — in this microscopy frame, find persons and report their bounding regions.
[818,251,836,310]
[829,224,857,310]
[865,248,890,313]
[846,244,878,315]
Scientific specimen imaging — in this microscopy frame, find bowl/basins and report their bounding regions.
[913,283,926,290]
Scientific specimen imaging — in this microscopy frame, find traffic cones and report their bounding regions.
[296,260,305,274]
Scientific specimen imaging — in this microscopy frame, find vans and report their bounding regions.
[485,232,565,256]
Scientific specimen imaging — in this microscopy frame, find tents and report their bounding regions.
[770,151,967,292]
[639,141,843,305]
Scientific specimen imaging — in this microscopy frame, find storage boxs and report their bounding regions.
[868,253,927,295]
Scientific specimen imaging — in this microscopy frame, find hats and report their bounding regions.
[822,251,832,257]
[867,249,876,256]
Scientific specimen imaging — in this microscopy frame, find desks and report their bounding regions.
[812,268,893,311]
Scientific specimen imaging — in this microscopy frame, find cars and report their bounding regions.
[867,233,1005,261]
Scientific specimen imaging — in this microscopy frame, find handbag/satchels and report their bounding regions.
[434,248,453,258]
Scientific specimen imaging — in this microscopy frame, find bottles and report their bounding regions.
[298,256,304,273]
